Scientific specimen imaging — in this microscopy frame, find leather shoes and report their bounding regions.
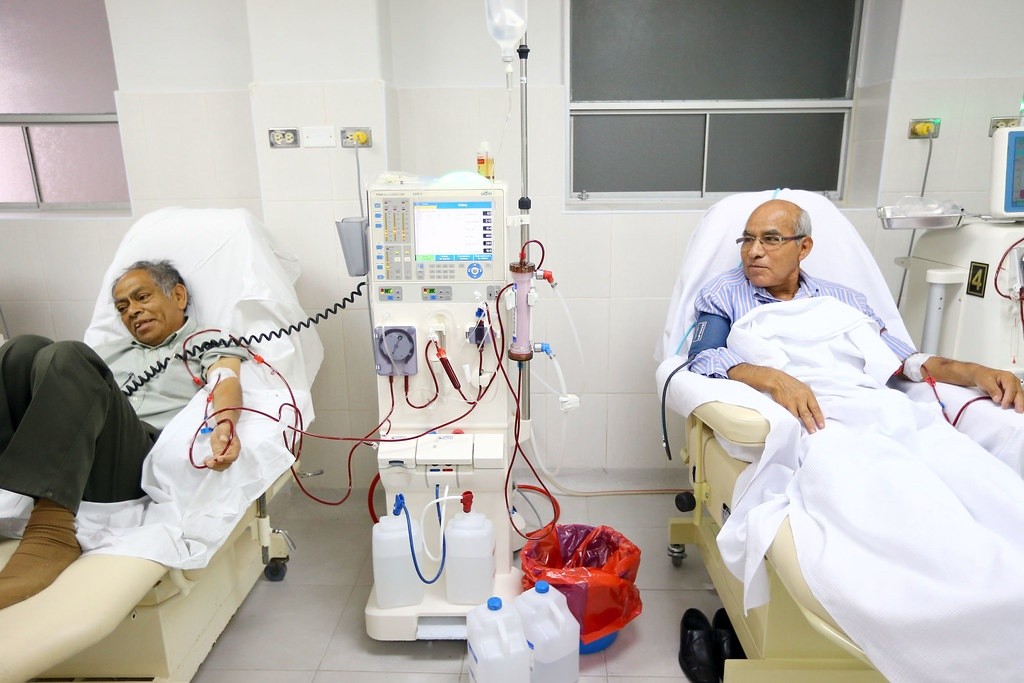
[712,607,748,683]
[678,607,724,683]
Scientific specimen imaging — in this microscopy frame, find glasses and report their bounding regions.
[735,234,806,251]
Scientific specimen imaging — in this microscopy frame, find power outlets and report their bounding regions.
[341,126,373,148]
[269,127,300,149]
[906,118,940,141]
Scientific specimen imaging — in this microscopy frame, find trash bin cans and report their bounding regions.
[519,524,644,656]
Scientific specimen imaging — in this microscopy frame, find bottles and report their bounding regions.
[467,597,531,683]
[477,142,495,184]
[516,580,580,683]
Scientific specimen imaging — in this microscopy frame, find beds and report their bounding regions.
[653,188,1024,683]
[0,206,324,683]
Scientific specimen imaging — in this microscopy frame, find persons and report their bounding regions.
[687,199,1023,574]
[1,260,250,609]
